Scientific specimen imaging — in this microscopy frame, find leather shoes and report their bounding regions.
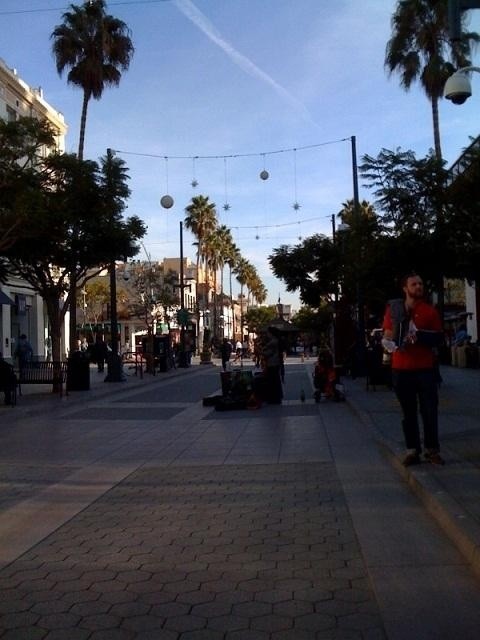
[402,454,422,467]
[425,451,445,465]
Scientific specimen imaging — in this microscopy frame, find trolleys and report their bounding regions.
[312,347,348,404]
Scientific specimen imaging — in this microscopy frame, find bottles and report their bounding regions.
[382,335,392,365]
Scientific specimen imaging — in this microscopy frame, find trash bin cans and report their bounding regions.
[365,328,391,393]
[67,351,90,392]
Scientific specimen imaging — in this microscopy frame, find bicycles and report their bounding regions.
[122,351,159,377]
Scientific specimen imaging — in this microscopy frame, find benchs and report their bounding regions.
[0,360,70,408]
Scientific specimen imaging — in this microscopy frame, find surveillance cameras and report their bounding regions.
[338,224,351,238]
[445,73,472,105]
[123,272,130,281]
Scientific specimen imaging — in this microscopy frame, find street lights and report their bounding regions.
[443,65,480,106]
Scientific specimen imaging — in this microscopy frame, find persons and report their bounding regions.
[219,326,288,384]
[12,333,34,380]
[1,352,19,407]
[452,323,467,346]
[379,268,448,468]
